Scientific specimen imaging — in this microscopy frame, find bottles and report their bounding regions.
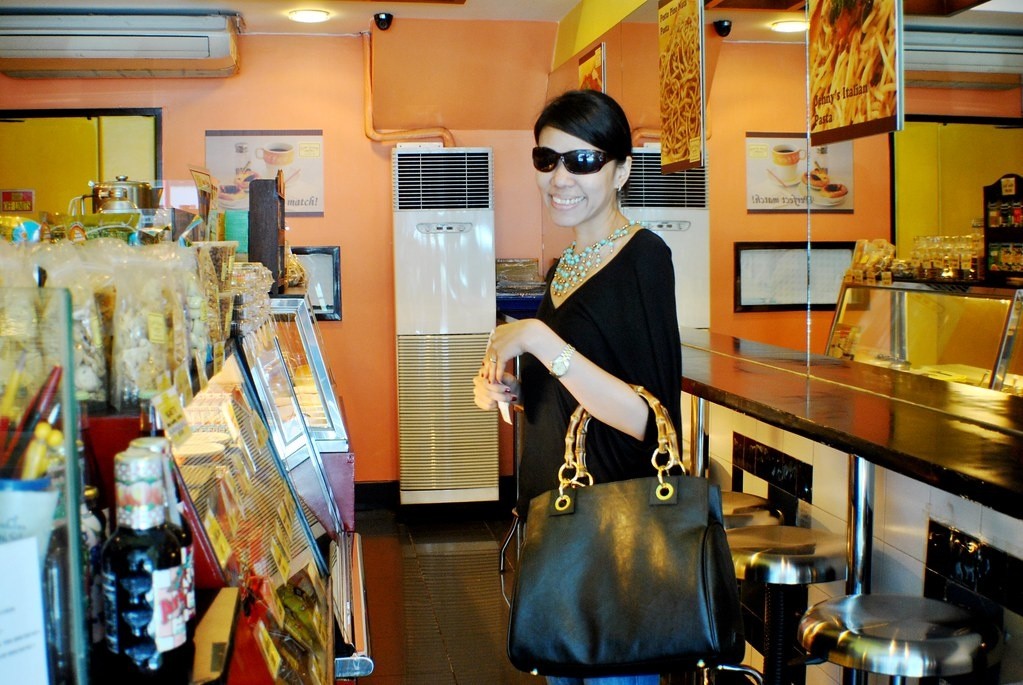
[910,234,985,281]
[234,142,250,192]
[38,428,201,685]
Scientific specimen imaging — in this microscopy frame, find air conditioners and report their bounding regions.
[621,145,712,331]
[387,142,504,505]
[0,0,246,80]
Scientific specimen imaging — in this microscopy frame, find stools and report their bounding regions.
[717,490,983,685]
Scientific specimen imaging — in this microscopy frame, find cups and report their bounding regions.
[770,144,806,181]
[767,143,806,182]
[254,143,297,179]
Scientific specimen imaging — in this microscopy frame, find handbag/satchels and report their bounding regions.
[506,379,747,677]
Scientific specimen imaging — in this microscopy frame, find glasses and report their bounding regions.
[532,146,612,175]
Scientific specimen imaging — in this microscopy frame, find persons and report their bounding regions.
[471,89,684,685]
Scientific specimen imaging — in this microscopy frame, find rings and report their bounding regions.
[489,358,496,363]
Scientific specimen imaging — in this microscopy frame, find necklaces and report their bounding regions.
[552,220,647,297]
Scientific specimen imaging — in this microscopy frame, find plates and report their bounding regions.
[216,195,250,209]
[265,165,305,186]
[763,166,804,186]
[801,173,822,190]
[812,194,848,206]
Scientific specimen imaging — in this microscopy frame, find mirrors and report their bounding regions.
[547,0,1022,401]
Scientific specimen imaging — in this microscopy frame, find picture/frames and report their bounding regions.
[732,240,869,311]
[290,246,343,322]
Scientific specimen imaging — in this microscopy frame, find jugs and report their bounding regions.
[79,175,163,228]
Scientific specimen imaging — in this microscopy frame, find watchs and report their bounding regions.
[549,344,575,377]
